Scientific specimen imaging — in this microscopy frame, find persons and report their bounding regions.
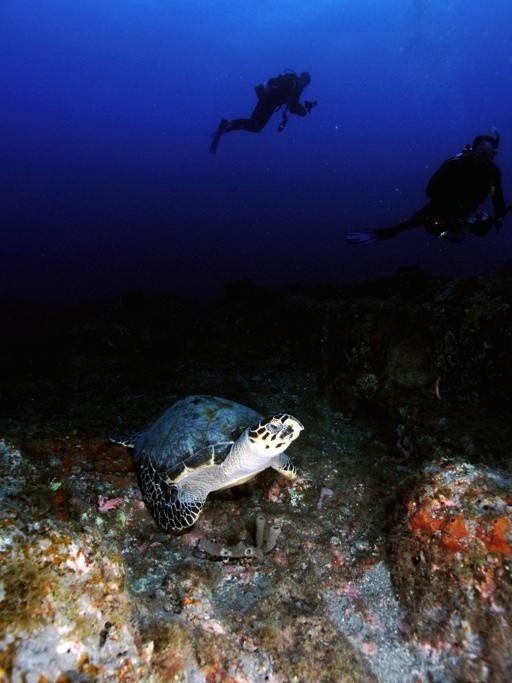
[208,70,313,156]
[343,132,506,250]
